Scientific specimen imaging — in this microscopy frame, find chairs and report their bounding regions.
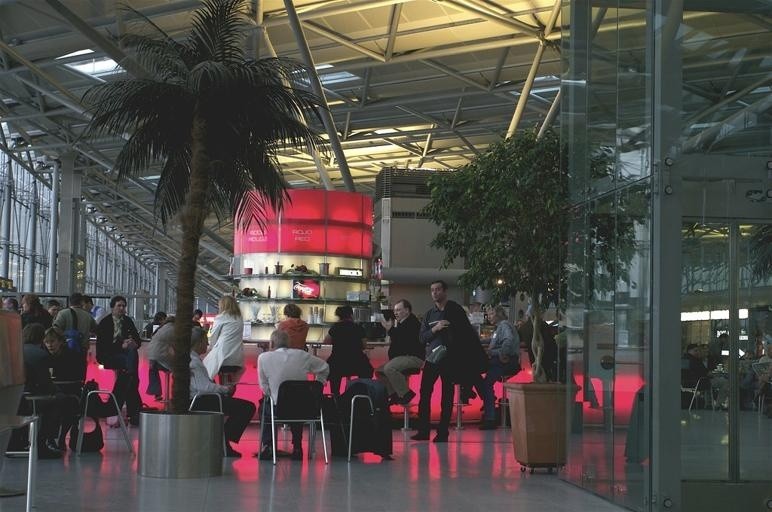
[187,392,231,459]
[258,379,329,466]
[20,383,136,464]
[328,379,395,463]
[680,358,771,415]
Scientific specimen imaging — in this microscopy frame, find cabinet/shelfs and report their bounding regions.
[214,269,379,330]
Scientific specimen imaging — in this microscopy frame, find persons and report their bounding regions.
[380,281,565,442]
[680,333,771,410]
[0,281,141,458]
[141,293,374,460]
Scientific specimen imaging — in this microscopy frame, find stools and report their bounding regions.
[0,415,43,512]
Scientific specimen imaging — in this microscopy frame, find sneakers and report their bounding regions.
[433,434,448,442]
[410,432,429,440]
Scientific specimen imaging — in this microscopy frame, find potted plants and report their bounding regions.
[71,0,348,479]
[409,121,654,476]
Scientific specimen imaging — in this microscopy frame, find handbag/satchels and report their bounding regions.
[69,416,104,452]
[64,329,80,350]
